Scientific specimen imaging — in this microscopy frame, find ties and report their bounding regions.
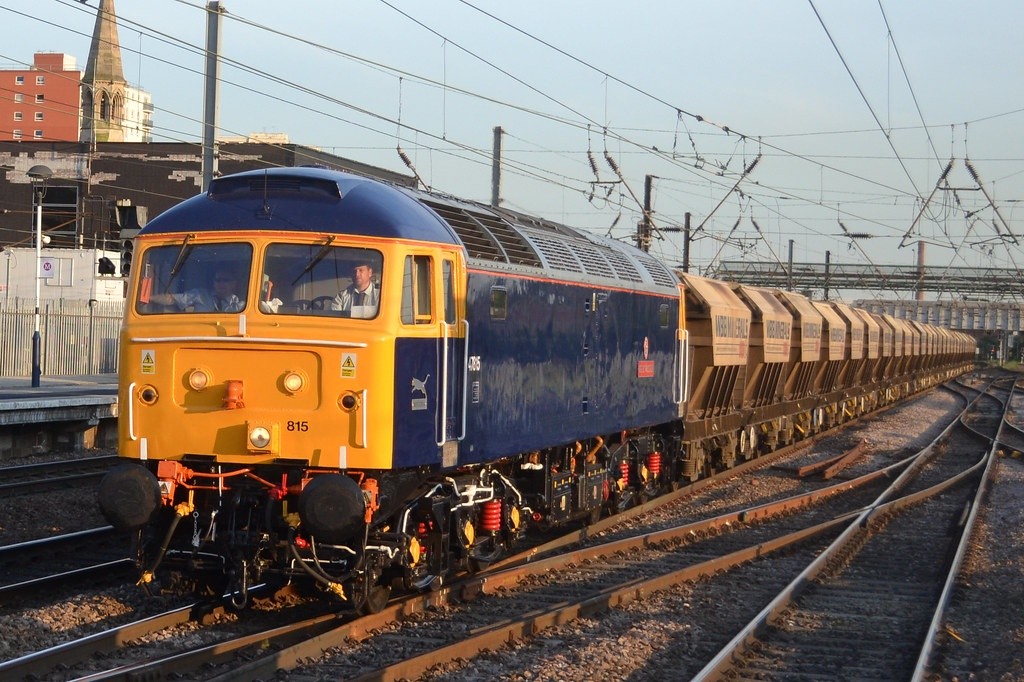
[355,292,366,306]
[215,295,225,312]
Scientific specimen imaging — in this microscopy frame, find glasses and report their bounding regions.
[213,277,234,283]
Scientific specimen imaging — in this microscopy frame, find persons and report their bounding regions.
[330,261,381,321]
[150,265,272,313]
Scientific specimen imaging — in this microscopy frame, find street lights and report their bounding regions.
[24,165,55,390]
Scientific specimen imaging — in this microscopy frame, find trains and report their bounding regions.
[111,162,980,617]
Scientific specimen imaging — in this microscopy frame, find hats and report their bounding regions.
[349,257,375,268]
